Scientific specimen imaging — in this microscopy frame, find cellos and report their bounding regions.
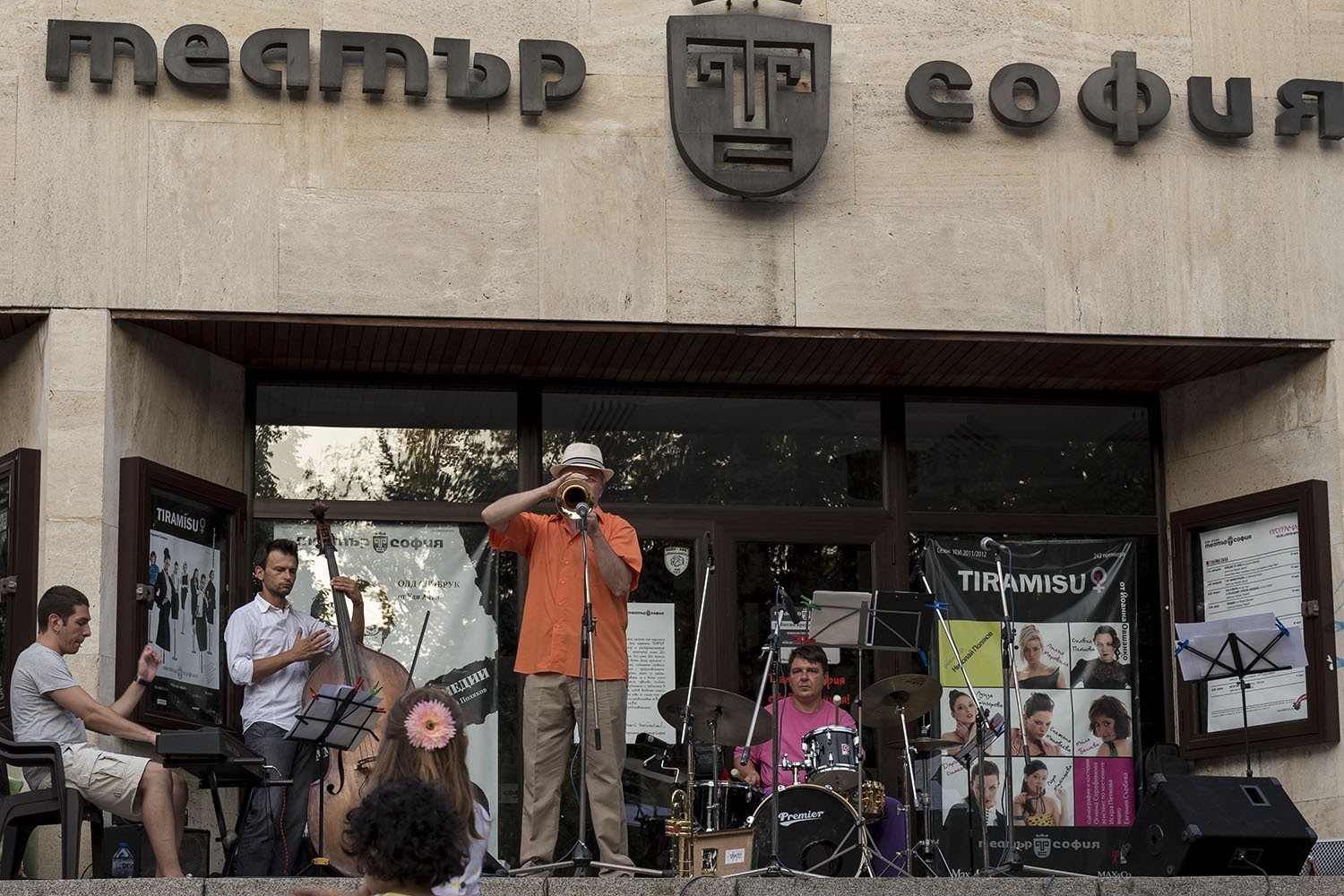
[297,496,419,873]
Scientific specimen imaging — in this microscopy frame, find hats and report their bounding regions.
[550,442,615,482]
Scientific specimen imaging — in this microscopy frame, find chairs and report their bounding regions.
[0,723,103,880]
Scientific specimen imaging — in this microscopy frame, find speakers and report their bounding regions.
[104,823,211,878]
[1114,773,1318,877]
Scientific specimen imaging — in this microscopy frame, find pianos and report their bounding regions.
[156,725,297,879]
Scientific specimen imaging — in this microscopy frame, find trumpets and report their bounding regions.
[555,478,597,521]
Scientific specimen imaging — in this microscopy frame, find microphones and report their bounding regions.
[575,502,589,517]
[706,531,716,574]
[909,540,930,582]
[778,586,800,623]
[980,537,1009,552]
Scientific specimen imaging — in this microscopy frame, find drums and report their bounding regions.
[846,777,887,822]
[694,778,755,831]
[745,782,869,878]
[800,723,865,791]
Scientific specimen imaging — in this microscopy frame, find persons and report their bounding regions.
[945,760,1007,826]
[9,585,191,878]
[942,690,993,755]
[1009,692,1062,756]
[1088,696,1132,756]
[481,442,643,878]
[149,547,216,673]
[1013,760,1069,826]
[297,776,472,896]
[366,688,490,896]
[732,643,866,875]
[1010,624,1066,688]
[223,539,364,877]
[1070,625,1131,689]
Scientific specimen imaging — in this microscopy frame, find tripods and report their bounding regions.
[496,516,675,879]
[284,687,378,877]
[979,550,1096,878]
[720,602,956,881]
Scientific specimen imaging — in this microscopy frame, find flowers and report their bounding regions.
[406,700,457,751]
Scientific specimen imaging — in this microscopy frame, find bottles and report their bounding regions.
[111,842,135,878]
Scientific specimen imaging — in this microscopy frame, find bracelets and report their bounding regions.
[1014,816,1024,820]
[134,676,151,687]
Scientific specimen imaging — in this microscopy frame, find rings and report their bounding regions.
[315,645,319,648]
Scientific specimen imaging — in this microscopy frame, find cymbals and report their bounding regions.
[656,685,772,747]
[852,674,943,729]
[882,735,964,754]
